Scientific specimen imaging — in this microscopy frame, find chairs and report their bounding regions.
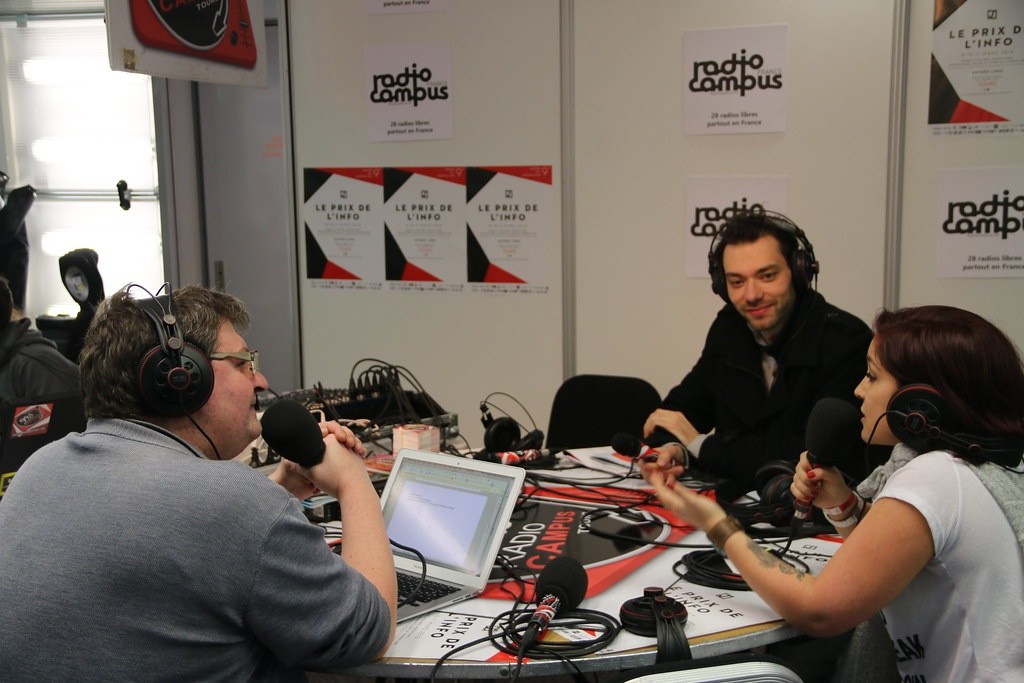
[547,372,656,455]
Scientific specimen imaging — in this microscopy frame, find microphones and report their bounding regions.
[259,399,326,470]
[472,448,565,468]
[611,433,679,469]
[791,396,861,534]
[519,555,589,649]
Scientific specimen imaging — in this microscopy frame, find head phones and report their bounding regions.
[618,586,693,665]
[886,382,1024,468]
[708,209,818,305]
[480,391,545,451]
[715,458,796,528]
[125,281,214,419]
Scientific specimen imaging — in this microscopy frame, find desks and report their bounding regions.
[307,457,845,683]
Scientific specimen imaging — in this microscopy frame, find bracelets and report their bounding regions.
[823,490,865,528]
[706,515,744,551]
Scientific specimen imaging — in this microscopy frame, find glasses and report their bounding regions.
[209,350,258,375]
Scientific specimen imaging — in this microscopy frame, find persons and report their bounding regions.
[640,211,894,489]
[0,278,398,683]
[642,304,1024,683]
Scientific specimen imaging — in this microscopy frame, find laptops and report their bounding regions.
[332,448,527,623]
